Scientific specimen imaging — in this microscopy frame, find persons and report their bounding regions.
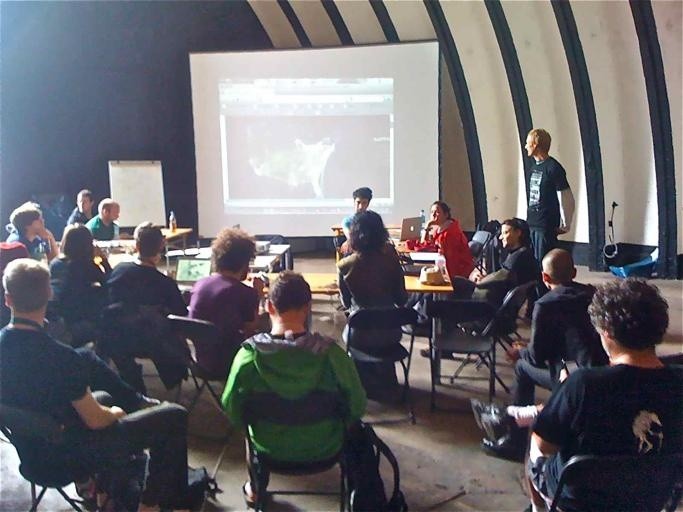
[1,259,187,511]
[471,276,682,510]
[221,269,367,508]
[337,208,407,397]
[402,218,538,360]
[524,128,574,326]
[340,188,372,258]
[0,189,120,266]
[189,229,272,382]
[480,248,610,462]
[108,220,192,376]
[406,200,472,315]
[50,224,112,325]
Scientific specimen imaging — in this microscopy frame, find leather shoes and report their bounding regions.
[480,435,527,463]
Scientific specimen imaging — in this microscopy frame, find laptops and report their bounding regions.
[394,216,422,243]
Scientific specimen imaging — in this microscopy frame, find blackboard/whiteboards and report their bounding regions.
[108,158,167,229]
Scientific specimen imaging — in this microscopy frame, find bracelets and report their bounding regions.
[424,227,433,231]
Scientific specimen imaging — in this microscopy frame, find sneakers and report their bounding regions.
[242,478,269,507]
[470,397,518,444]
[420,347,457,361]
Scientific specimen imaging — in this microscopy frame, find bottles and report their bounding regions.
[168,210,178,232]
[419,209,428,229]
